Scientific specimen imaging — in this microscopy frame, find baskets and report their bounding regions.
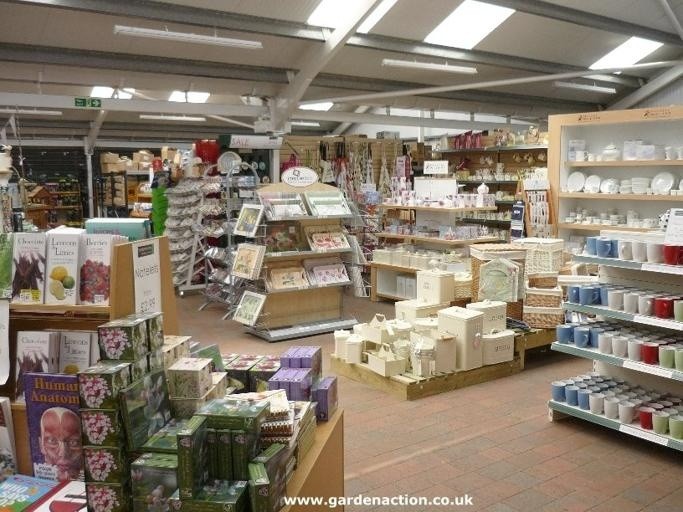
[469,241,528,321]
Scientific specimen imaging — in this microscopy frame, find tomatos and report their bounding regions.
[80,259,111,302]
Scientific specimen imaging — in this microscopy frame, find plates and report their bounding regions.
[567,171,683,195]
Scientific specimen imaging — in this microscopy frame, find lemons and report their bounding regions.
[50,280,64,300]
[63,276,74,289]
[50,267,68,282]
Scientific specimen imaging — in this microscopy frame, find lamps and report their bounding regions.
[381,55,477,76]
[553,79,616,95]
[112,18,262,52]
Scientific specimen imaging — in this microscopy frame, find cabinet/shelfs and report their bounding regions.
[0,105,683,511]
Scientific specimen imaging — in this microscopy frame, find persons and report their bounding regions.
[38,407,82,482]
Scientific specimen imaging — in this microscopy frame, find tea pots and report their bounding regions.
[602,142,621,161]
[476,182,489,194]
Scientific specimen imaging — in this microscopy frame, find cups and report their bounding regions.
[586,236,683,266]
[455,152,547,182]
[556,316,683,372]
[551,373,683,439]
[576,151,602,161]
[382,176,478,209]
[565,206,659,228]
[568,282,683,321]
